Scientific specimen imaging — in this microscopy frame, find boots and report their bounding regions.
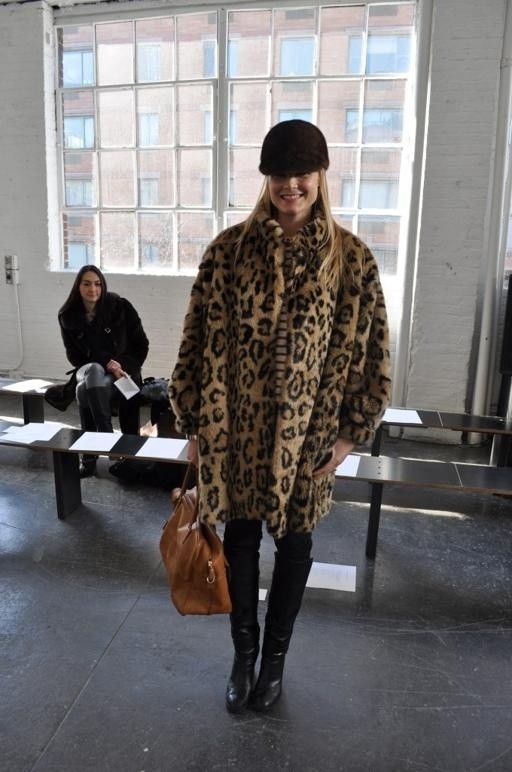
[78,386,114,479]
[222,540,260,714]
[250,551,315,710]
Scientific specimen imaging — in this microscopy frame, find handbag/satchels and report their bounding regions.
[160,462,233,617]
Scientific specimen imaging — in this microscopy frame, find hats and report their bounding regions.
[258,118,330,176]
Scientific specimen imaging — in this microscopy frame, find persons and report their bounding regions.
[164,118,395,714]
[58,265,150,479]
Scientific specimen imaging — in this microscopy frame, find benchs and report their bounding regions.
[3,374,508,467]
[3,421,509,560]
